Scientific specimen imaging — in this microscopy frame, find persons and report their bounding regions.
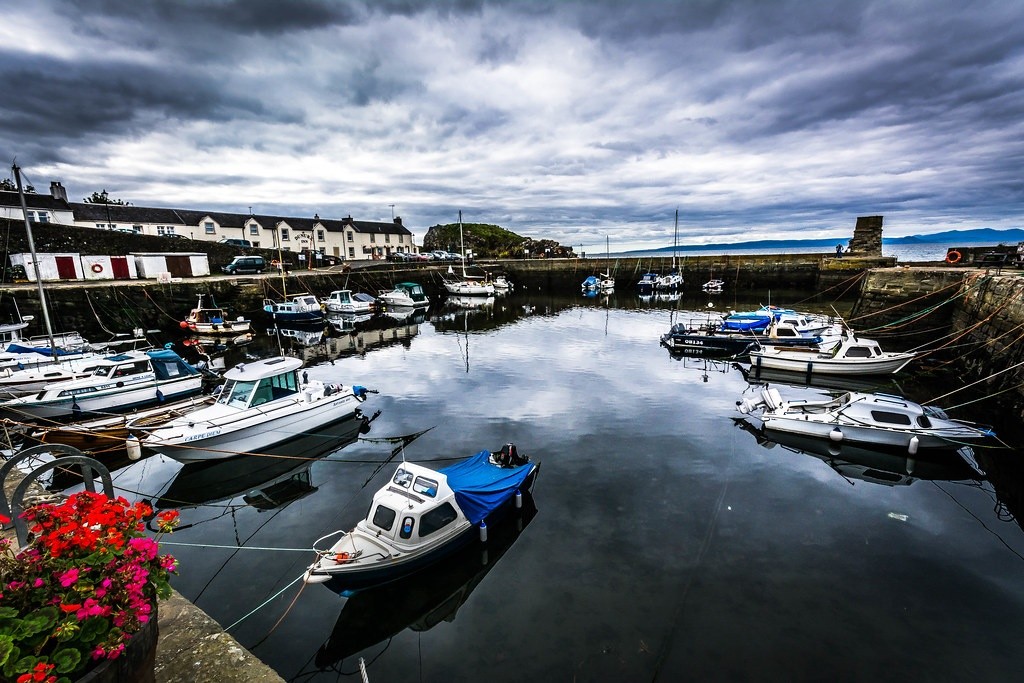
[1017,241,1024,262]
[277,261,282,276]
[834,243,843,259]
[282,260,288,275]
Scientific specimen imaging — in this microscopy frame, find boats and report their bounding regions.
[139,410,380,533]
[638,273,661,291]
[582,275,601,289]
[494,276,508,288]
[731,416,975,486]
[303,442,541,591]
[0,281,429,462]
[702,279,724,288]
[314,490,537,669]
[660,288,918,374]
[735,383,993,448]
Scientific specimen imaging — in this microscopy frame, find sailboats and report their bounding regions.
[0,165,205,423]
[657,210,684,289]
[600,235,615,288]
[442,211,495,296]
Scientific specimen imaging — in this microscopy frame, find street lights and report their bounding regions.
[101,190,111,229]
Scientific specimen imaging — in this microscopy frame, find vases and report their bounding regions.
[71,582,160,683]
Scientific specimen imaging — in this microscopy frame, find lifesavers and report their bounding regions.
[192,312,196,319]
[324,331,329,336]
[92,264,103,272]
[946,251,961,264]
[321,304,325,309]
[481,281,484,284]
[541,253,544,257]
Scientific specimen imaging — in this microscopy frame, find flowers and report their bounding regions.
[0,492,181,683]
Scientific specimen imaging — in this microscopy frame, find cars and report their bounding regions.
[115,228,466,274]
